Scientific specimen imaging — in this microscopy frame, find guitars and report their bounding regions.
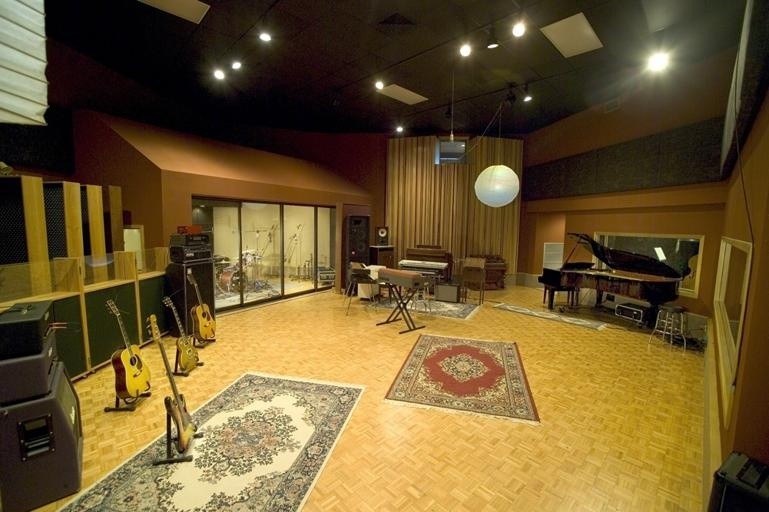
[146,315,197,453]
[106,299,150,404]
[187,273,217,342]
[162,297,199,373]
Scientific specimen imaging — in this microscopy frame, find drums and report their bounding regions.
[219,272,248,293]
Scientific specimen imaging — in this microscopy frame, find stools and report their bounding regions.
[647,304,689,352]
[342,269,377,316]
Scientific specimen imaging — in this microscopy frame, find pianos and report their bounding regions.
[347,262,377,284]
[538,233,684,327]
[378,268,424,289]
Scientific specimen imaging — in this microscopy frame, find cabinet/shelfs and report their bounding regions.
[369,245,395,269]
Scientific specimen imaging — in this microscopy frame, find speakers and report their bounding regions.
[345,215,371,266]
[375,226,389,247]
[169,261,216,337]
[0,360,87,511]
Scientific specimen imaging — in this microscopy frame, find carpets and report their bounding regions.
[55,370,368,512]
[385,333,541,424]
[492,301,608,331]
[368,296,479,320]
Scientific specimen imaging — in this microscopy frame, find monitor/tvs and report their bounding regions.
[438,139,467,161]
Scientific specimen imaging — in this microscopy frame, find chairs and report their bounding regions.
[461,255,487,305]
[349,261,387,303]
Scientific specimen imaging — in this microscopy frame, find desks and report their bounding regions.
[378,282,402,304]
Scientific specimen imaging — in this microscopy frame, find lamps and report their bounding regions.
[474,99,521,208]
